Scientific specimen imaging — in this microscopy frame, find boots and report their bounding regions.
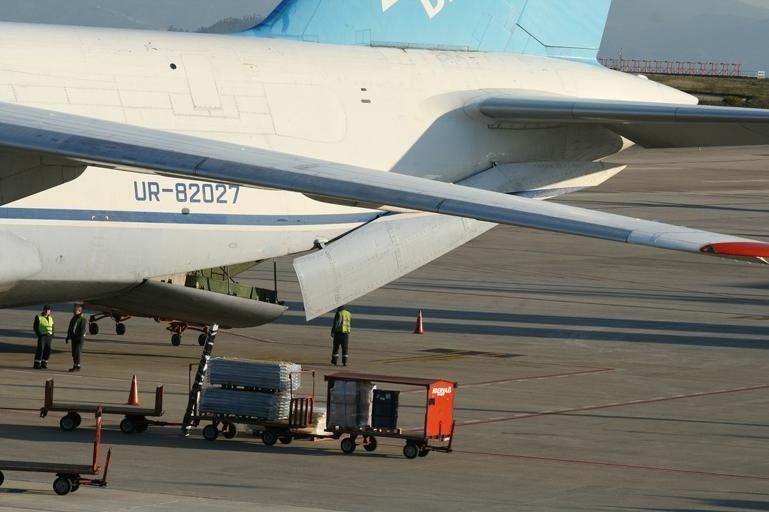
[70,364,80,372]
[331,354,348,366]
[34,360,48,369]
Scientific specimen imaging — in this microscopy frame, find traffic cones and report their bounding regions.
[413,308,427,336]
[125,371,141,407]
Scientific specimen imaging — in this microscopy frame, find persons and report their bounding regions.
[65,304,87,373]
[31,304,55,370]
[330,306,352,366]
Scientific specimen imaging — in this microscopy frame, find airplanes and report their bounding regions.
[0,1,769,344]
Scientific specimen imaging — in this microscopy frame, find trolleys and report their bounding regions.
[1,408,115,495]
[44,365,458,459]
